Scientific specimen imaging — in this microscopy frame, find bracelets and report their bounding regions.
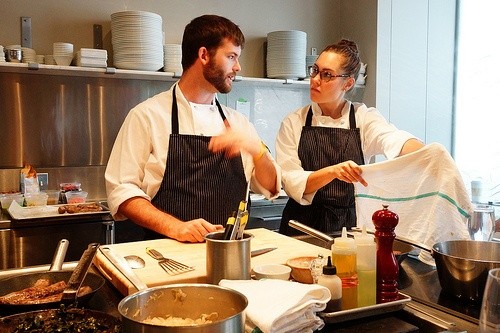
[252,145,267,160]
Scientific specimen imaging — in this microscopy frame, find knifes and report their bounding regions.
[251,247,277,257]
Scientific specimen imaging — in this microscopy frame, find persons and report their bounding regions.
[105,15,281,244]
[275,39,466,239]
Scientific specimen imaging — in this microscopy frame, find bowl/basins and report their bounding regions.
[0,182,88,210]
[252,264,292,281]
[286,257,326,284]
[53,42,74,66]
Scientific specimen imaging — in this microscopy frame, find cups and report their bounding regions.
[479,268,500,333]
[467,205,496,241]
[203,232,254,286]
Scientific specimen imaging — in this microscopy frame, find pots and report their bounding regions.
[351,225,500,305]
[0,243,123,333]
[99,244,249,333]
[0,239,106,318]
[288,220,415,267]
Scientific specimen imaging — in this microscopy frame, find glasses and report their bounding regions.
[308,65,350,82]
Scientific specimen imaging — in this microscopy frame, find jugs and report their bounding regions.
[356,61,368,85]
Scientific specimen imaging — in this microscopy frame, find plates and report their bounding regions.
[111,10,183,73]
[6,46,57,65]
[266,30,320,80]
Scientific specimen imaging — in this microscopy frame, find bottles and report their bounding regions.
[317,255,343,313]
[331,224,377,311]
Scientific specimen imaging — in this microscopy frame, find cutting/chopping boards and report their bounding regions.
[90,224,331,300]
